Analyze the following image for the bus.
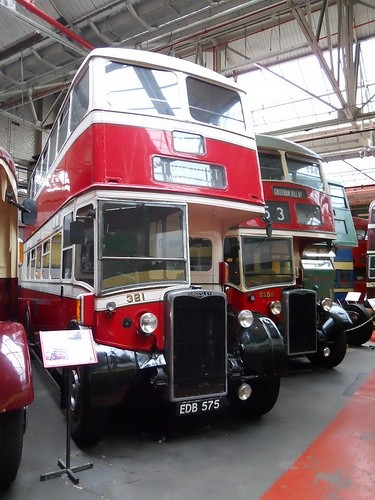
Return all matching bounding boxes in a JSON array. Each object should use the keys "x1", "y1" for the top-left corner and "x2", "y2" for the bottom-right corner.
[
  {"x1": 0, "y1": 145, "x2": 34, "y2": 493},
  {"x1": 15, "y1": 46, "x2": 284, "y2": 448},
  {"x1": 252, "y1": 133, "x2": 375, "y2": 369}
]
